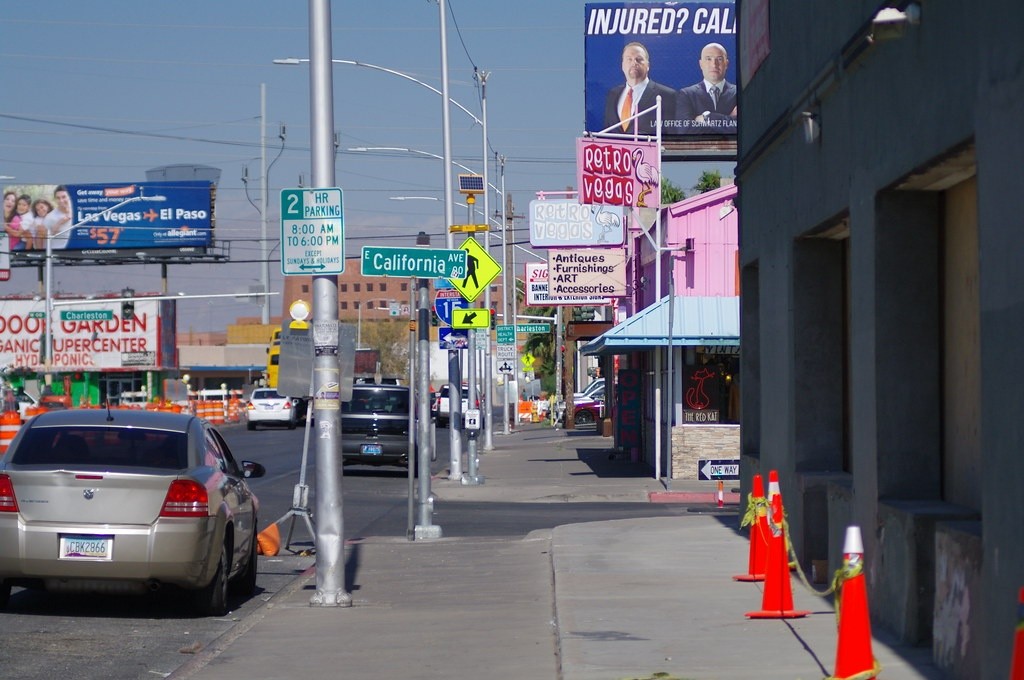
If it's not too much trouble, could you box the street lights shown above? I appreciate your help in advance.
[44,195,168,363]
[272,57,511,451]
[357,297,396,349]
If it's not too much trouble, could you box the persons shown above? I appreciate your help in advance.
[606,42,677,135]
[542,407,558,425]
[3,384,63,412]
[4,185,73,249]
[677,42,738,134]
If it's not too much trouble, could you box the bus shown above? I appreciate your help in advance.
[265,327,282,389]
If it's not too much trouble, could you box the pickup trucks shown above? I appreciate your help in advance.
[436,384,480,429]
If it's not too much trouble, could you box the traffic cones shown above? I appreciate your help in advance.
[743,494,811,618]
[732,471,797,581]
[833,527,876,680]
[1010,587,1024,680]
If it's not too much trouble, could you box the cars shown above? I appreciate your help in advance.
[561,401,605,424]
[0,410,268,615]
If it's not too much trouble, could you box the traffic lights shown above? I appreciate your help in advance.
[587,306,596,320]
[574,308,582,321]
[491,307,497,330]
[432,306,438,326]
[562,345,565,368]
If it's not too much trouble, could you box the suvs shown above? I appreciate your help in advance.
[544,378,606,422]
[245,387,299,430]
[342,383,419,478]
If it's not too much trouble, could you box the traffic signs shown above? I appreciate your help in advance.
[697,458,741,482]
[281,187,346,276]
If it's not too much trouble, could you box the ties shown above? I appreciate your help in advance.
[710,86,719,111]
[620,89,633,132]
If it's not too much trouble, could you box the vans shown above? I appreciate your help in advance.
[356,378,401,412]
